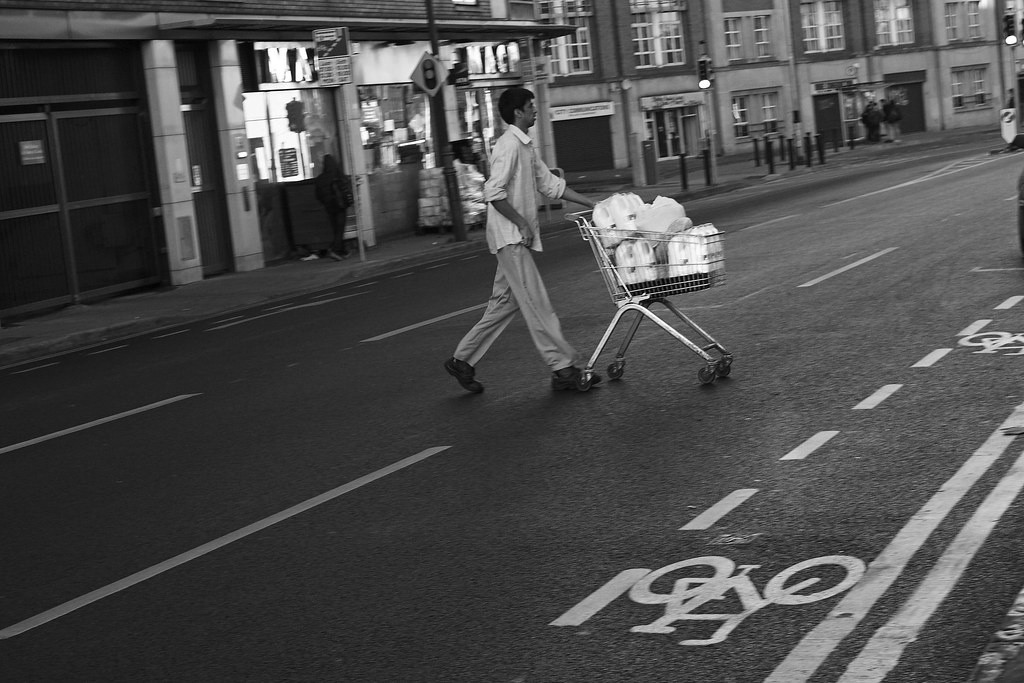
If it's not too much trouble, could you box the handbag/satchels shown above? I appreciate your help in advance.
[346,192,354,207]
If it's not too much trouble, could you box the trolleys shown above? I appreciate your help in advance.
[563,204,736,396]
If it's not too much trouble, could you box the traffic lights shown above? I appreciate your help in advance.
[411,51,450,98]
[706,59,717,84]
[1020,18,1024,37]
[1007,15,1016,37]
[697,59,708,89]
[1003,15,1010,38]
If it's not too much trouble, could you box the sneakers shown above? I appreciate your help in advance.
[444,357,484,391]
[552,369,603,391]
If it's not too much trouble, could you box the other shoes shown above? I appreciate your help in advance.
[331,252,344,260]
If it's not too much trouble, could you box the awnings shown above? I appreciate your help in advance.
[158,14,578,40]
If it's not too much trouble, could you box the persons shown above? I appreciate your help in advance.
[1008,89,1015,108]
[861,99,900,142]
[445,89,602,391]
[315,154,350,259]
